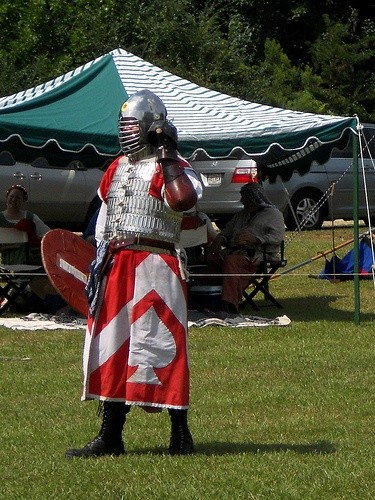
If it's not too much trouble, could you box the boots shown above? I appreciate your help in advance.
[66,402,127,457]
[169,408,194,454]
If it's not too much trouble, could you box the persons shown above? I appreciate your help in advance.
[213,181,284,314]
[65,88,202,458]
[180,209,222,266]
[84,208,97,249]
[0,184,60,301]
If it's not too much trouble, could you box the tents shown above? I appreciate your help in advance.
[0,47,361,326]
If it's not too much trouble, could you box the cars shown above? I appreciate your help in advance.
[260,122,375,232]
[0,151,261,236]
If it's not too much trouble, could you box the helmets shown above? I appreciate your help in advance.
[117,89,168,157]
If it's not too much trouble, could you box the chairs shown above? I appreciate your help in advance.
[0,219,288,316]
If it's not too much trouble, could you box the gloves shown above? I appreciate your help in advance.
[148,119,177,160]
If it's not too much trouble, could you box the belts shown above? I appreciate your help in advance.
[135,237,175,252]
